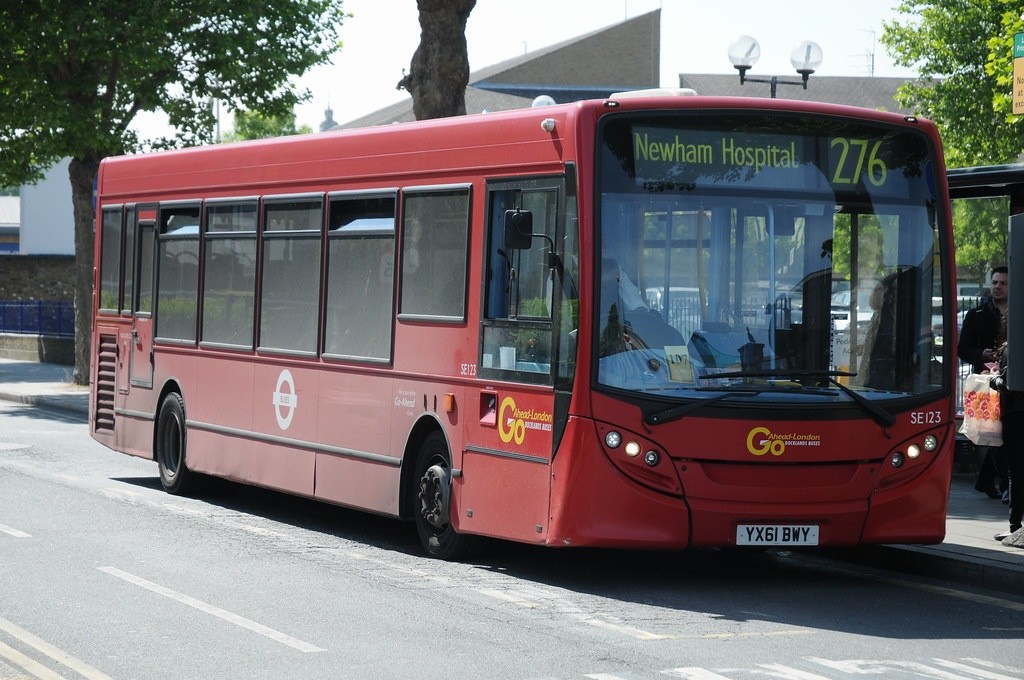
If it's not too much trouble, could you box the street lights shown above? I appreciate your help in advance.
[726,33,824,98]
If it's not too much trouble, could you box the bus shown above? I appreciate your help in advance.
[88,91,959,560]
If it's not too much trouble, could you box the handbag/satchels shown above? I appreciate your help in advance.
[958,374,1002,446]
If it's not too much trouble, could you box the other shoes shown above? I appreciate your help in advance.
[1002,490,1009,504]
[995,529,1012,540]
[974,479,1003,498]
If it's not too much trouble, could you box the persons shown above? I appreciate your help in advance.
[957,267,1024,541]
[865,272,896,391]
[545,224,650,318]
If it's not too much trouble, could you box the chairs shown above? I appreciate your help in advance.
[167,250,239,267]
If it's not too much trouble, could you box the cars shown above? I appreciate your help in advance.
[644,283,981,383]
